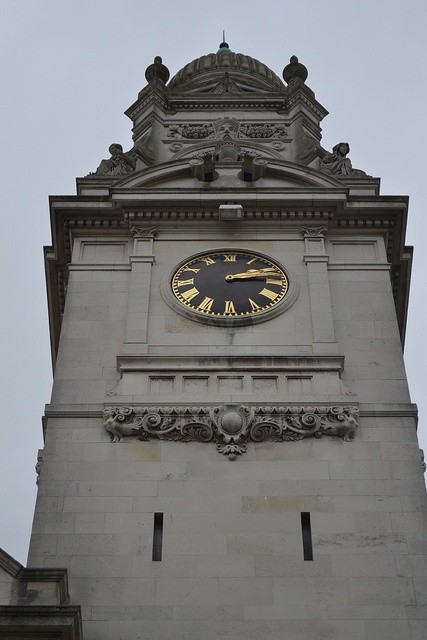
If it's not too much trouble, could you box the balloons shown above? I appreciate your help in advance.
[159,247,300,327]
